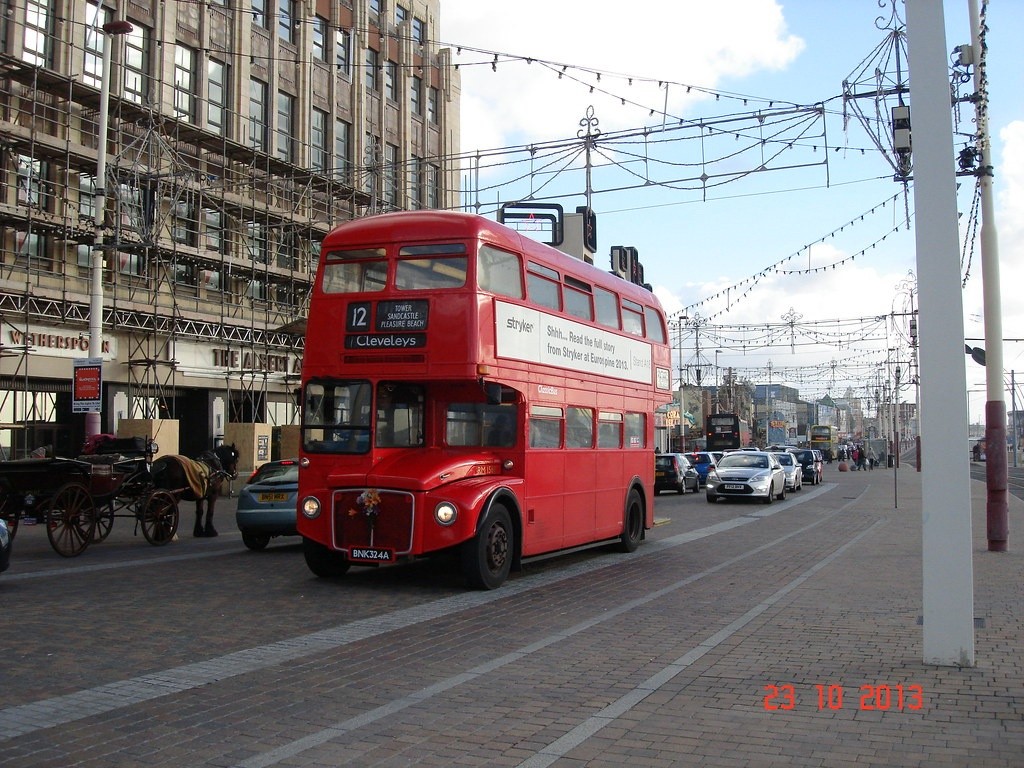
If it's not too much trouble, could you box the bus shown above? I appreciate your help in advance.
[297,200,670,592]
[809,425,839,462]
[706,413,750,450]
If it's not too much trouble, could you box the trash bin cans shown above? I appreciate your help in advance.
[888,453,895,468]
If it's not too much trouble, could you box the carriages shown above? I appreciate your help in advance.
[1,435,240,560]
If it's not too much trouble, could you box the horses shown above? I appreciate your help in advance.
[151,441,239,539]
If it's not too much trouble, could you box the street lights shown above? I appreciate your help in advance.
[678,315,689,452]
[888,346,900,466]
[765,386,778,448]
[715,349,724,414]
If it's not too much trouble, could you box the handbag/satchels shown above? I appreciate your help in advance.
[865,459,869,466]
[874,460,879,466]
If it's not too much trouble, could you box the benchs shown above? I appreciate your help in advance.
[0,457,93,482]
[78,453,124,478]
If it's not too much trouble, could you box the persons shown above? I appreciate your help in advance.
[825,445,877,472]
[655,447,661,454]
[487,408,617,448]
[694,445,700,452]
[972,444,979,462]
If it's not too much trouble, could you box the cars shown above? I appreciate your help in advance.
[713,443,824,486]
[681,453,718,487]
[237,457,299,550]
[705,451,787,505]
[770,452,804,493]
[653,451,701,495]
